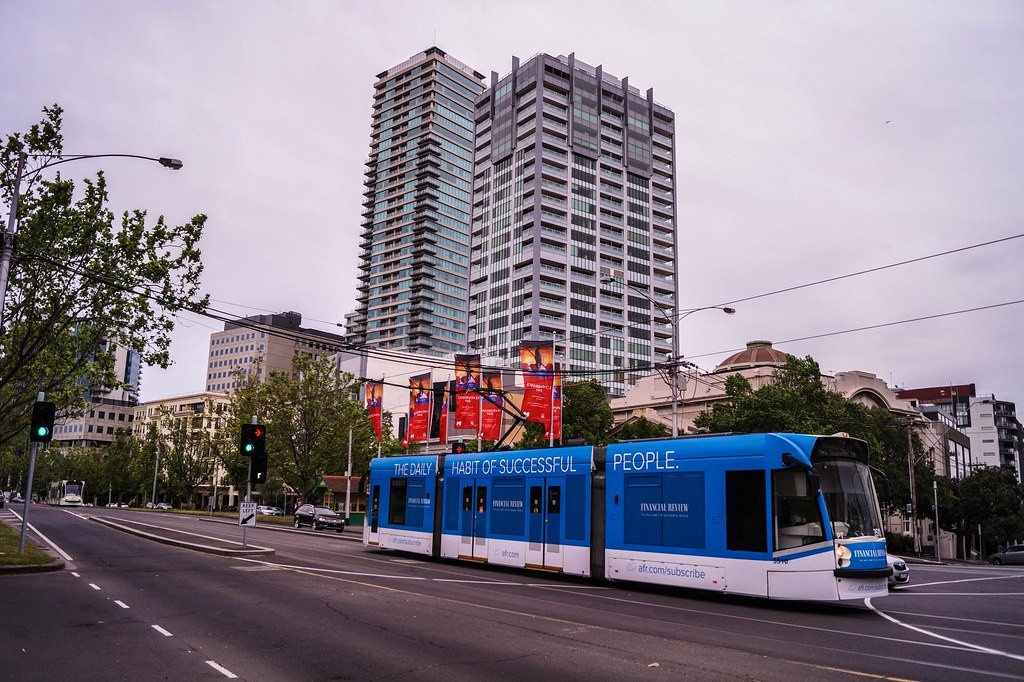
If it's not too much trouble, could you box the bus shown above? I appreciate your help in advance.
[362,431,889,601]
[47,479,84,506]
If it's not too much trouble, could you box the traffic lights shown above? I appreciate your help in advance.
[30,402,56,442]
[241,425,266,457]
[452,442,467,453]
[251,455,266,483]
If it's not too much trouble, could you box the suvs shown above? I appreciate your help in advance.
[294,504,344,533]
[0,490,5,508]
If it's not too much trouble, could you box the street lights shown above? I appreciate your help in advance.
[0,154,183,324]
[599,277,736,436]
[147,438,159,502]
[909,399,996,558]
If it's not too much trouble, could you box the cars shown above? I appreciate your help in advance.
[256,505,284,517]
[106,503,115,507]
[115,501,129,508]
[156,503,172,510]
[988,544,1024,566]
[146,502,156,508]
[887,554,910,587]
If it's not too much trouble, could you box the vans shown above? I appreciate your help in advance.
[11,497,25,504]
